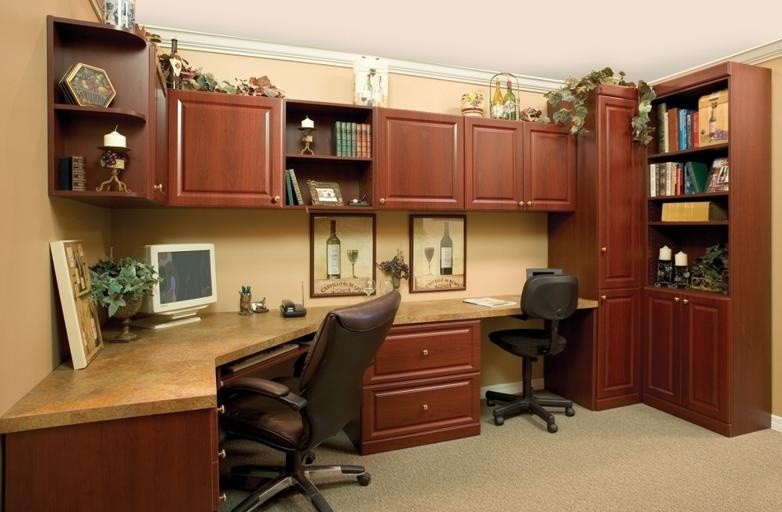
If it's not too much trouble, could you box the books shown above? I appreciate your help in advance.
[367,124,371,158]
[686,162,708,194]
[286,170,295,206]
[677,169,684,195]
[362,124,367,158]
[659,103,666,153]
[357,124,362,157]
[659,163,666,196]
[290,169,305,206]
[650,164,657,198]
[687,114,692,149]
[694,111,699,148]
[684,166,695,195]
[656,163,659,196]
[334,121,342,156]
[347,123,351,157]
[341,122,347,157]
[668,108,679,152]
[664,112,669,153]
[463,297,517,309]
[352,123,357,157]
[354,58,389,107]
[672,163,684,196]
[679,109,697,151]
[666,162,672,196]
[691,116,694,148]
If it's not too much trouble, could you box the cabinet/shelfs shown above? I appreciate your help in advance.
[463,116,578,213]
[47,14,168,209]
[547,84,648,411]
[642,61,774,438]
[167,87,465,212]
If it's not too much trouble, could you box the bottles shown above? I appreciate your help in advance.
[326,220,341,279]
[708,110,717,138]
[439,221,453,275]
[504,80,518,121]
[164,38,182,90]
[490,81,506,121]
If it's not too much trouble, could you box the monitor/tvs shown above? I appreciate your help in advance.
[133,240,218,330]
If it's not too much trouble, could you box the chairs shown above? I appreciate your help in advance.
[215,288,402,512]
[484,274,579,433]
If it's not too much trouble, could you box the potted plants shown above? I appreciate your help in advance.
[89,255,164,345]
[378,250,409,289]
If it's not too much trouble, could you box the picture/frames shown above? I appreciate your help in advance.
[307,179,344,206]
[408,214,467,294]
[309,212,376,299]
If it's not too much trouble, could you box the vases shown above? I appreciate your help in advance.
[461,108,485,118]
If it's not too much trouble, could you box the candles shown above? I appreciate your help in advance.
[674,250,688,266]
[658,245,672,260]
[300,115,314,129]
[103,123,126,147]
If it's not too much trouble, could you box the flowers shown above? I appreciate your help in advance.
[460,92,485,108]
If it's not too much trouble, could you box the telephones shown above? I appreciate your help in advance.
[279,280,307,318]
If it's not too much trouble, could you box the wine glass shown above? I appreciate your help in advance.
[424,247,435,277]
[362,279,378,302]
[380,279,392,295]
[347,249,359,278]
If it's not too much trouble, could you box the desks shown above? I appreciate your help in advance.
[0,293,599,512]
